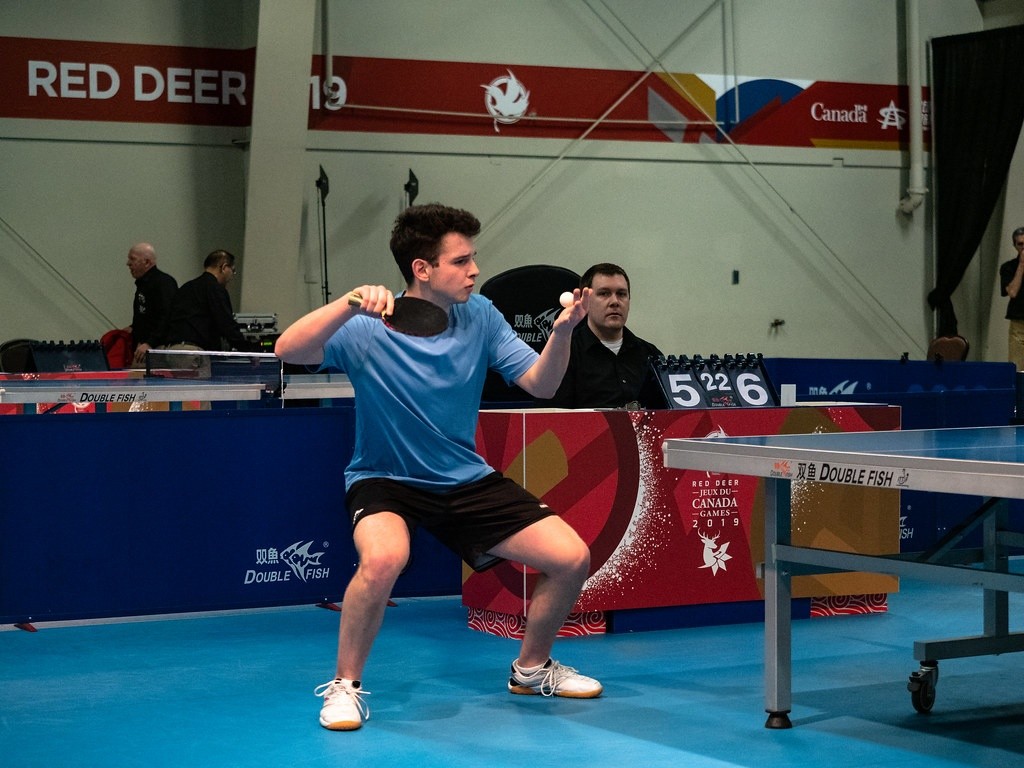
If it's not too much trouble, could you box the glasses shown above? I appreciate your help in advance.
[220,263,236,275]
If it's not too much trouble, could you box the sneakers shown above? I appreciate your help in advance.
[313,677,372,731]
[507,656,603,700]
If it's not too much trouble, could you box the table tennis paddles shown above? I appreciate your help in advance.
[346,293,452,337]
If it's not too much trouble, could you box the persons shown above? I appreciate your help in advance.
[998,226,1024,375]
[177,249,267,378]
[118,242,180,370]
[551,262,672,408]
[272,200,604,733]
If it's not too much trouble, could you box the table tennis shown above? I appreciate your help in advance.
[561,291,575,308]
